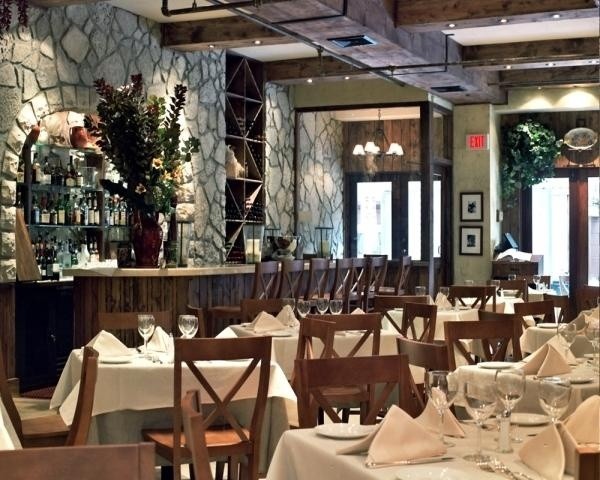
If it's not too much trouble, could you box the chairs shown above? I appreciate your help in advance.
[291,354,407,428]
[185,306,203,335]
[98,310,172,341]
[273,254,600,379]
[0,402,157,480]
[239,298,280,319]
[3,375,71,448]
[208,260,279,329]
[141,334,273,478]
[396,335,470,422]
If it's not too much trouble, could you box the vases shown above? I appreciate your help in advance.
[133,217,162,269]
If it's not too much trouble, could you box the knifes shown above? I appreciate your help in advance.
[364,455,454,471]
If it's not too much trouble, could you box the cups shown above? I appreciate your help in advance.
[508,274,517,281]
[539,377,574,419]
[177,312,199,338]
[414,285,426,296]
[295,297,346,318]
[463,279,501,291]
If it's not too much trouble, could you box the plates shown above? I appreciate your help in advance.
[478,360,512,369]
[504,410,548,429]
[314,423,371,440]
[566,374,593,384]
[101,355,131,365]
[564,127,597,151]
[538,322,557,328]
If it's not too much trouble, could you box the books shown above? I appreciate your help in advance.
[496,248,532,263]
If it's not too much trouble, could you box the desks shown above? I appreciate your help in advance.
[48,343,300,478]
[222,317,411,405]
[450,359,599,421]
[265,417,598,480]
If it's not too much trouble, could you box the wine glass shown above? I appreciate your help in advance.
[137,314,155,351]
[282,297,297,331]
[464,374,497,466]
[439,286,450,311]
[495,367,531,444]
[532,274,542,291]
[422,366,456,450]
[557,318,600,372]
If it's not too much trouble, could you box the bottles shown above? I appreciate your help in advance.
[31,152,84,187]
[225,115,264,222]
[15,190,102,226]
[103,196,129,227]
[29,235,100,280]
[226,246,244,263]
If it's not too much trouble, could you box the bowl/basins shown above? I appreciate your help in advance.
[266,235,301,257]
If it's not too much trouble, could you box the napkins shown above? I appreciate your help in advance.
[83,330,131,360]
[518,424,581,478]
[245,311,281,330]
[335,405,445,468]
[146,327,173,351]
[561,393,598,446]
[213,328,237,340]
[415,396,465,440]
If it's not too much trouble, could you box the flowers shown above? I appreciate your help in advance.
[84,72,203,216]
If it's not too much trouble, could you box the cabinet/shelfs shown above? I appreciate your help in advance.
[224,47,267,264]
[24,143,133,277]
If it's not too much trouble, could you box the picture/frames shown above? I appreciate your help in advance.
[458,226,484,257]
[460,192,485,221]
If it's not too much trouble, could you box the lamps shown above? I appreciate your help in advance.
[350,107,405,156]
